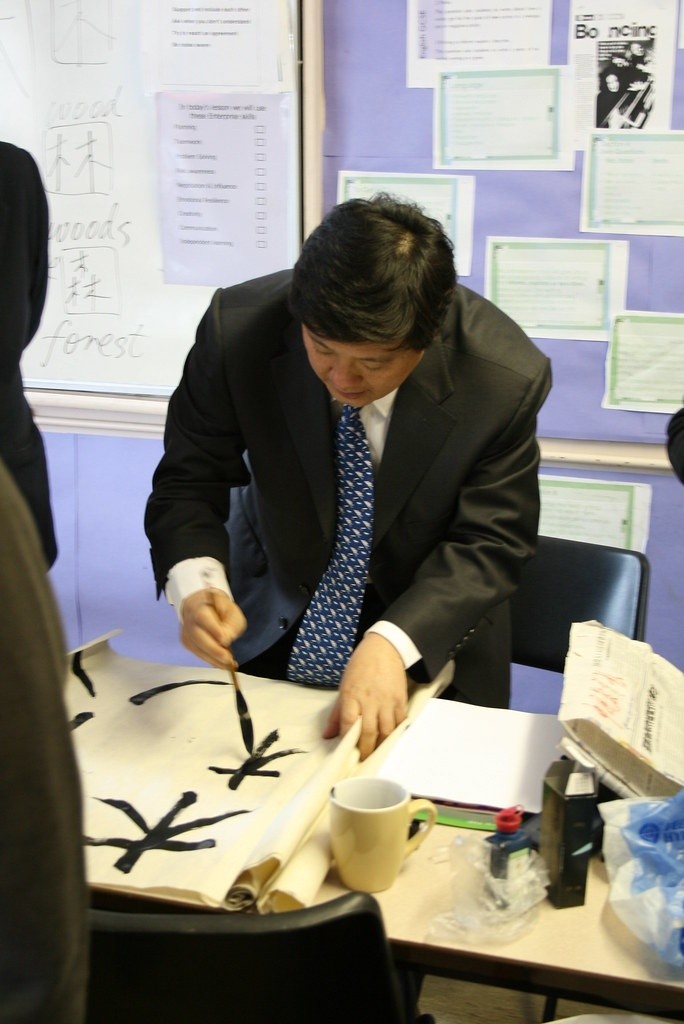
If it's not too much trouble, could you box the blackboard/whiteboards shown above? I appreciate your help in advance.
[0,0,327,422]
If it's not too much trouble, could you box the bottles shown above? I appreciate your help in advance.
[478,805,532,910]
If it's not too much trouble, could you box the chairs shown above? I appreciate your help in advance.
[83,889,436,1024]
[510,535,651,674]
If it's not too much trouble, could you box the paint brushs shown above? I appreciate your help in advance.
[201,570,254,756]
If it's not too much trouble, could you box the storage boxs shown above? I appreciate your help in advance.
[537,737,599,910]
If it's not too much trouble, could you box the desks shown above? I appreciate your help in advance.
[83,802,684,1024]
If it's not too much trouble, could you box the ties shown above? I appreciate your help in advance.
[287,404,373,688]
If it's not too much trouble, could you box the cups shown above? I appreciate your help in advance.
[330,778,438,894]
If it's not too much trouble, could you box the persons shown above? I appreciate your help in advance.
[0,140,58,574]
[595,41,656,131]
[0,463,87,1024]
[143,198,553,764]
